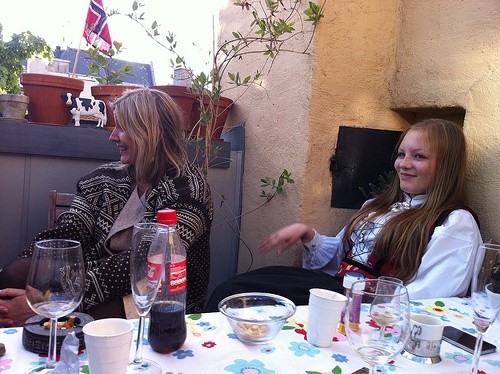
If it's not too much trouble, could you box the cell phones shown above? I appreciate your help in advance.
[442,326,497,356]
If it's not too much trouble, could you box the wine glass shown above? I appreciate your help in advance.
[130,223,169,374]
[25,239,85,374]
[345,276,411,374]
[460,242,500,374]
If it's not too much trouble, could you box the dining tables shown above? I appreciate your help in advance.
[0,296,500,374]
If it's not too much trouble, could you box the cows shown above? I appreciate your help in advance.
[60,91,108,129]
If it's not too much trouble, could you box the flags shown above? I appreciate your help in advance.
[84,0,112,54]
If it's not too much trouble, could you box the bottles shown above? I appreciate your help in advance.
[146,209,187,354]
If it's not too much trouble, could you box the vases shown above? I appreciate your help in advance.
[18,72,83,126]
[148,85,232,141]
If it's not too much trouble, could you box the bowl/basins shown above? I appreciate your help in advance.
[218,292,296,344]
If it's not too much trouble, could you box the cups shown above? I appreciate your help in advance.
[307,288,348,347]
[82,318,133,374]
[404,314,444,357]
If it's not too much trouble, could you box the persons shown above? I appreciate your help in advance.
[1,87,214,328]
[206,119,486,312]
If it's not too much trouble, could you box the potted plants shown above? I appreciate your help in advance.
[0,18,54,125]
[87,44,145,130]
